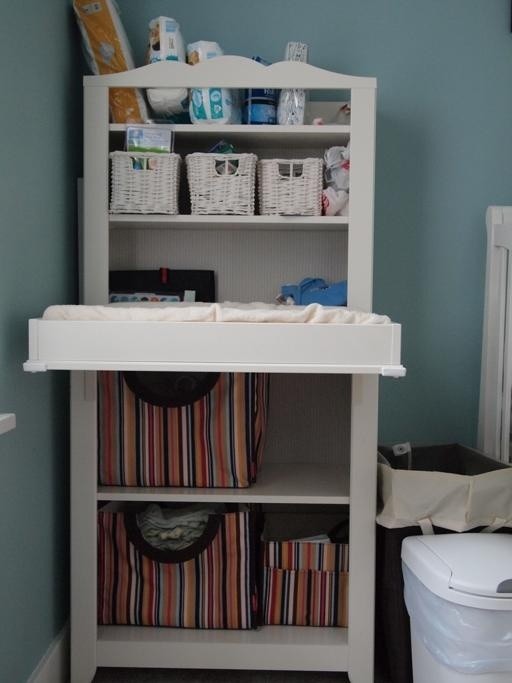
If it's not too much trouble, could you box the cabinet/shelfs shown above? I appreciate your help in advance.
[26,58,402,683]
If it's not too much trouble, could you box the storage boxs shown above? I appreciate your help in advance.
[260,537,348,627]
[99,501,264,634]
[99,371,268,489]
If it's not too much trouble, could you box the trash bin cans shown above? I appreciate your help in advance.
[401,533,512,683]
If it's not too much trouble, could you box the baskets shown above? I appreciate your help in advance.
[260,157,324,217]
[112,150,179,215]
[187,152,255,214]
